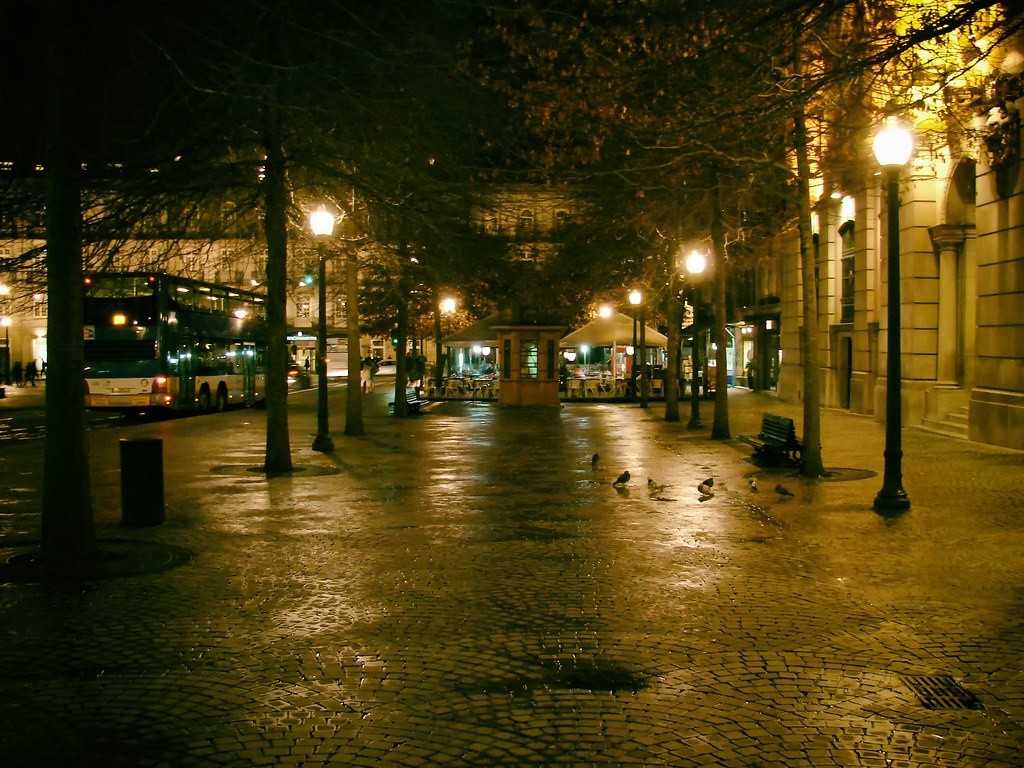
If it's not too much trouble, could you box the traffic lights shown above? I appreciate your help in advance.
[305,276,313,284]
[392,337,399,345]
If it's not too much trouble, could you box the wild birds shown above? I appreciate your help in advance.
[704,478,714,487]
[591,454,599,465]
[698,483,714,495]
[648,477,657,489]
[613,471,630,485]
[649,485,665,499]
[748,475,757,491]
[776,484,795,498]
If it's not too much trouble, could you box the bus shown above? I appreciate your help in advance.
[82,269,265,416]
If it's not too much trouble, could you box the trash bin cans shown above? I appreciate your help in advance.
[120,438,165,524]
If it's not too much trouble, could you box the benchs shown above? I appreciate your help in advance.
[737,412,822,467]
[388,390,430,413]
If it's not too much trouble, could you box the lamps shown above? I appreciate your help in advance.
[625,342,634,356]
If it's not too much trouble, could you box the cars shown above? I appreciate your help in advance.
[286,362,311,389]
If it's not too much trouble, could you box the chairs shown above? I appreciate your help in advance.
[420,377,501,400]
[565,379,664,399]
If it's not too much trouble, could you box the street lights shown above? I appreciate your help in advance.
[2,318,12,385]
[308,206,335,451]
[685,249,707,431]
[869,114,914,516]
[444,298,455,377]
[629,291,641,402]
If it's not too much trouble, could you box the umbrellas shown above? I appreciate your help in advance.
[559,311,666,346]
[435,305,513,348]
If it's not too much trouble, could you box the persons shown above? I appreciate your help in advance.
[562,358,653,397]
[303,356,310,372]
[406,352,427,387]
[12,358,40,388]
[359,349,393,395]
